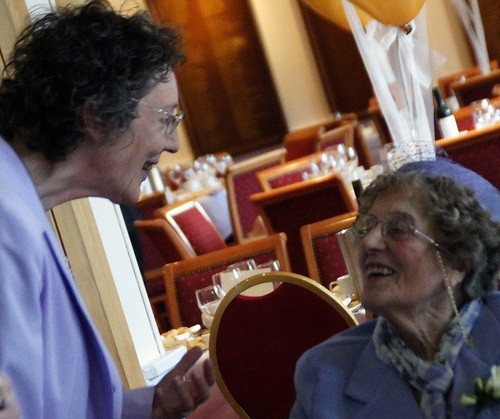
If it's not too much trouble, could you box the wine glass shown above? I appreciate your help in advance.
[168,155,232,200]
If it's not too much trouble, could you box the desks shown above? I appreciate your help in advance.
[174,175,232,241]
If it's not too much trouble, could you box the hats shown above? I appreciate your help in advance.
[396,158,500,224]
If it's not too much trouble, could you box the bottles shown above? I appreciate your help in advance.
[352,179,364,197]
[433,86,459,139]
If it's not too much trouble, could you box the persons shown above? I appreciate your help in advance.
[288,168,500,419]
[0,0,216,419]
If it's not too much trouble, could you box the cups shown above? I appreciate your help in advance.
[472,98,500,128]
[194,259,281,315]
[329,273,356,295]
[303,143,362,192]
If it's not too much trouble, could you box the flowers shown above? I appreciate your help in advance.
[462,365,500,419]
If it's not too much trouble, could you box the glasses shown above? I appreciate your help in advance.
[129,96,185,136]
[353,211,459,263]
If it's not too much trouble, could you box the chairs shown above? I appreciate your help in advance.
[125,61,500,419]
